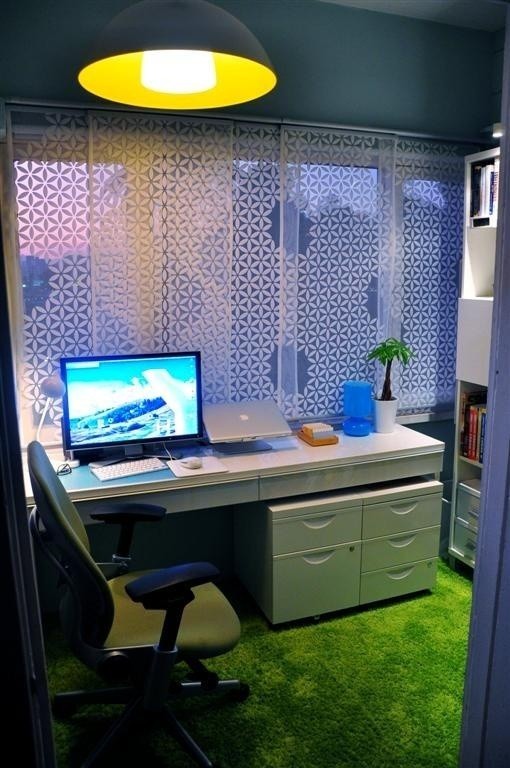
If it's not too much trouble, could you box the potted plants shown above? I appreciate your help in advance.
[367,337,414,434]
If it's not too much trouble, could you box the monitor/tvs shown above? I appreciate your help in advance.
[60,351,203,468]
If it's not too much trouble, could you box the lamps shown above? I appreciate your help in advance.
[75,1,278,110]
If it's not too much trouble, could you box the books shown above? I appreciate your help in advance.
[459,392,487,463]
[471,158,500,217]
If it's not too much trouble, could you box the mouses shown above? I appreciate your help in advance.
[180,457,202,469]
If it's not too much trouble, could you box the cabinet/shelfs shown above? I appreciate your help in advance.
[447,147,501,570]
[360,483,444,604]
[272,500,364,624]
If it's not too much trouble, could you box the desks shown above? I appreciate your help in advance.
[21,422,444,530]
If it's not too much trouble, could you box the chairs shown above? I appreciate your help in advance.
[26,440,249,768]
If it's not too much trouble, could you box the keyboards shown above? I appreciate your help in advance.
[89,456,170,483]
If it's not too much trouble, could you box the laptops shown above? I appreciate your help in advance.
[202,399,293,444]
